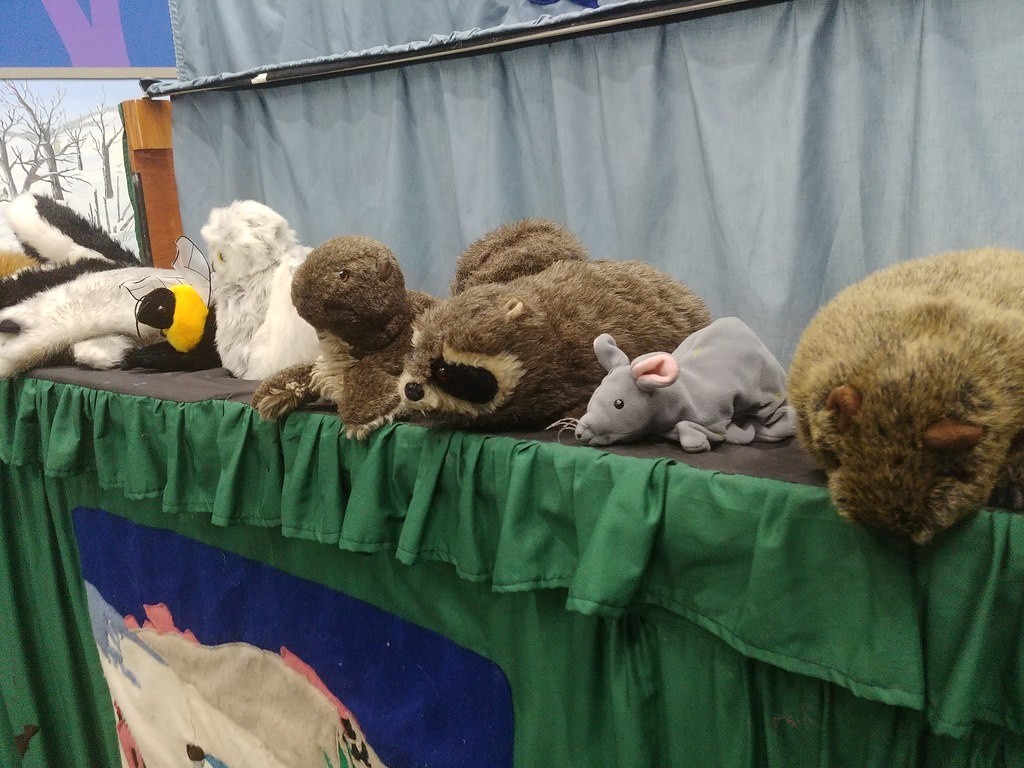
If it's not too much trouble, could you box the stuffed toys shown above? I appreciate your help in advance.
[121,283,223,373]
[547,316,795,453]
[397,258,714,434]
[201,200,321,382]
[788,246,1023,546]
[0,193,219,381]
[252,219,583,441]
